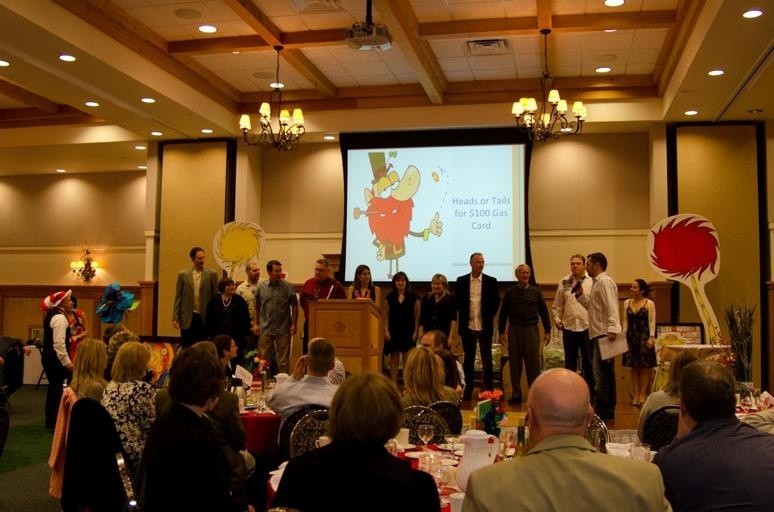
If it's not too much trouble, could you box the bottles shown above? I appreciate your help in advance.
[512,426,525,457]
[256,396,266,413]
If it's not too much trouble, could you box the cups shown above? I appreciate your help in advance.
[397,428,410,446]
[627,441,651,461]
[314,436,330,447]
[448,492,465,512]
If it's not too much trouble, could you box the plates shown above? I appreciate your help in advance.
[401,443,515,467]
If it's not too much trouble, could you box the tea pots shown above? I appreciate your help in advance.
[229,377,248,406]
[455,428,498,491]
[273,373,290,390]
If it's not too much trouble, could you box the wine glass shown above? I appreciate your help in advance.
[444,433,459,460]
[419,425,433,449]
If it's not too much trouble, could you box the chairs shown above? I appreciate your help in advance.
[57,388,140,511]
[643,401,683,453]
[586,411,611,450]
[276,396,464,463]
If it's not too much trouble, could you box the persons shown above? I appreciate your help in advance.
[552,254,597,401]
[461,368,672,512]
[498,264,550,406]
[623,279,657,406]
[43,288,467,510]
[651,359,774,512]
[572,252,622,420]
[637,352,700,444]
[172,247,454,388]
[454,253,501,399]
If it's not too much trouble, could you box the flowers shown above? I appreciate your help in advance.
[92,281,141,334]
[477,387,507,436]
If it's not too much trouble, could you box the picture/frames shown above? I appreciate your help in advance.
[653,322,704,366]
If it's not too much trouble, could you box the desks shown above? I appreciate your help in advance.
[21,344,49,386]
[229,381,282,458]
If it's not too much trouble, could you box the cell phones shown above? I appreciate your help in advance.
[244,406,256,410]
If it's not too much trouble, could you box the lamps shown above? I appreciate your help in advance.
[235,45,307,153]
[68,245,98,283]
[507,26,587,142]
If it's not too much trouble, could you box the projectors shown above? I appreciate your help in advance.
[344,25,393,53]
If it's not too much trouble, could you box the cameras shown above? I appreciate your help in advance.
[224,376,242,388]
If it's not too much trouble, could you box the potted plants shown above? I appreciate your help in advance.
[717,294,759,394]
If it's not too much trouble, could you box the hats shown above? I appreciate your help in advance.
[40,289,72,313]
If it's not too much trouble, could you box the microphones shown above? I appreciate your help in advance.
[571,275,586,293]
[328,279,336,286]
